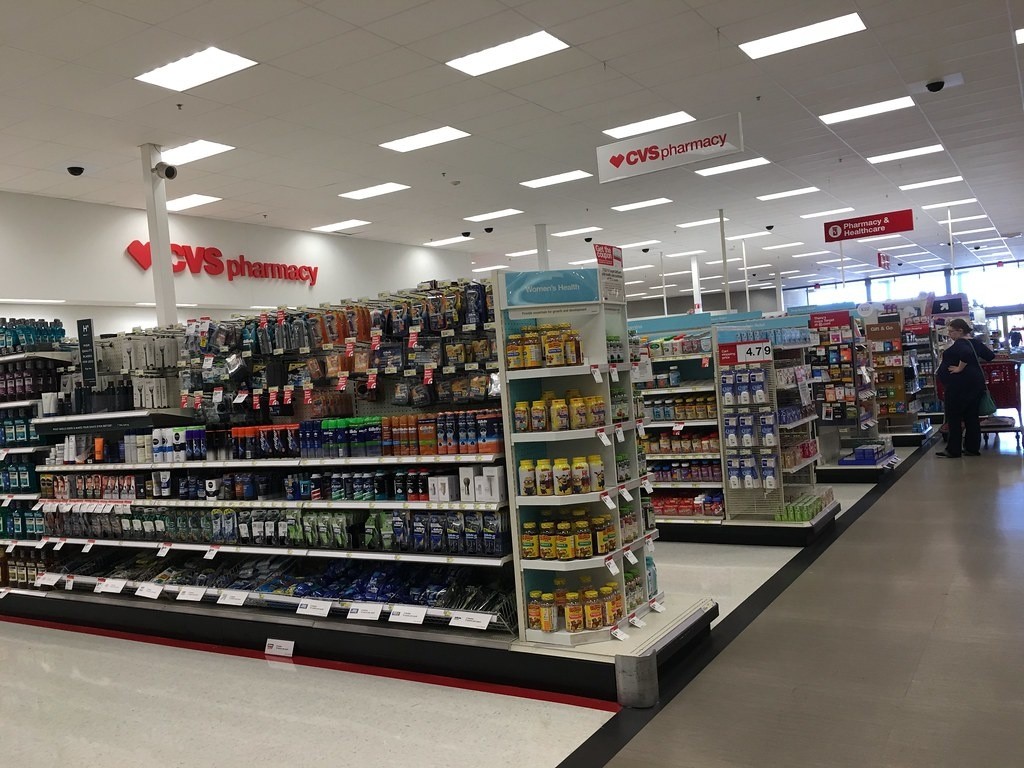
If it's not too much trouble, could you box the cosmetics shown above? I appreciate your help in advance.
[178,473,268,500]
[42,392,58,417]
[57,377,135,417]
[151,471,171,499]
[45,426,206,465]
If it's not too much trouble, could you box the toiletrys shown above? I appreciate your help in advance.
[0,317,516,636]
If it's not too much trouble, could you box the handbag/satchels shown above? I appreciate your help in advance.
[978,390,996,416]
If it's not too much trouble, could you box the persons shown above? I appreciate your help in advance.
[1008,326,1023,347]
[53,476,132,494]
[935,319,996,458]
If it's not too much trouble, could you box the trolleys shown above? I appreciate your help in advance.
[934,354,1024,448]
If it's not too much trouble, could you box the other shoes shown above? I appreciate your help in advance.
[935,452,951,458]
[961,449,981,456]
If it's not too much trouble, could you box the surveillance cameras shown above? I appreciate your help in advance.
[642,249,649,253]
[462,232,470,237]
[67,167,84,176]
[766,225,773,230]
[485,228,493,233]
[898,263,902,266]
[753,274,756,276]
[974,246,980,250]
[926,81,945,93]
[585,238,592,242]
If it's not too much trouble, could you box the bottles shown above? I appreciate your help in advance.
[522,506,615,560]
[553,577,570,616]
[643,396,722,483]
[514,387,606,434]
[518,454,606,497]
[283,465,448,500]
[118,408,502,461]
[578,575,592,598]
[620,496,657,547]
[565,592,584,632]
[528,590,542,630]
[615,444,647,484]
[606,330,642,363]
[601,586,617,626]
[624,556,658,615]
[606,582,624,619]
[541,593,558,633]
[507,320,585,374]
[611,387,644,422]
[585,590,603,631]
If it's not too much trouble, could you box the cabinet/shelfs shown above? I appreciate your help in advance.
[626,302,945,528]
[0,264,716,664]
